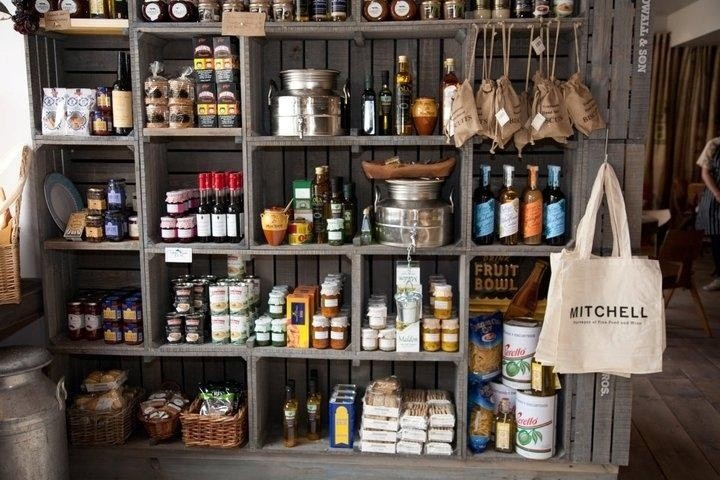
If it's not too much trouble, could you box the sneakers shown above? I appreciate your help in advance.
[702,279,720,291]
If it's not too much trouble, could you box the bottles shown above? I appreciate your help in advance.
[282,377,300,447]
[304,368,325,444]
[112,51,134,135]
[196,169,246,243]
[497,165,520,248]
[493,396,516,454]
[360,208,374,246]
[505,259,550,319]
[472,163,497,248]
[543,163,567,247]
[394,55,414,138]
[378,69,394,137]
[440,56,458,133]
[520,165,542,248]
[360,75,378,136]
[310,162,357,248]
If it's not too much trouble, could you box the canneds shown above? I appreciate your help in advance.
[362,274,458,351]
[66,293,137,348]
[420,0,575,18]
[87,186,138,242]
[289,221,312,235]
[515,388,558,460]
[326,218,344,245]
[289,235,311,245]
[89,88,111,135]
[197,0,294,23]
[502,316,538,390]
[253,273,348,350]
[163,255,261,343]
[160,188,200,239]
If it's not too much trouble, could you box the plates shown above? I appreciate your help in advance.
[42,171,86,235]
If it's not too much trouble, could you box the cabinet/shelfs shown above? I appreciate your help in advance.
[11,0,586,465]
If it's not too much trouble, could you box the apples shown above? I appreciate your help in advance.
[518,429,531,445]
[506,361,519,376]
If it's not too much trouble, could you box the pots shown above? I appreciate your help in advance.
[266,65,352,138]
[374,177,457,249]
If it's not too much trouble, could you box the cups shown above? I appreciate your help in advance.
[409,95,440,136]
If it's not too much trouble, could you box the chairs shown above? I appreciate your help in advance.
[657,228,714,339]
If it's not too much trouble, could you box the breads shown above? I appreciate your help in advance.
[138,389,190,419]
[70,370,133,413]
[146,75,194,129]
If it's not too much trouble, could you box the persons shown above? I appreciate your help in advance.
[695,138,720,292]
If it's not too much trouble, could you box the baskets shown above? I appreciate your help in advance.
[137,390,190,441]
[67,388,145,447]
[0,229,23,306]
[179,395,247,449]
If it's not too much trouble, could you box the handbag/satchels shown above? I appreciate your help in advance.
[532,162,667,380]
